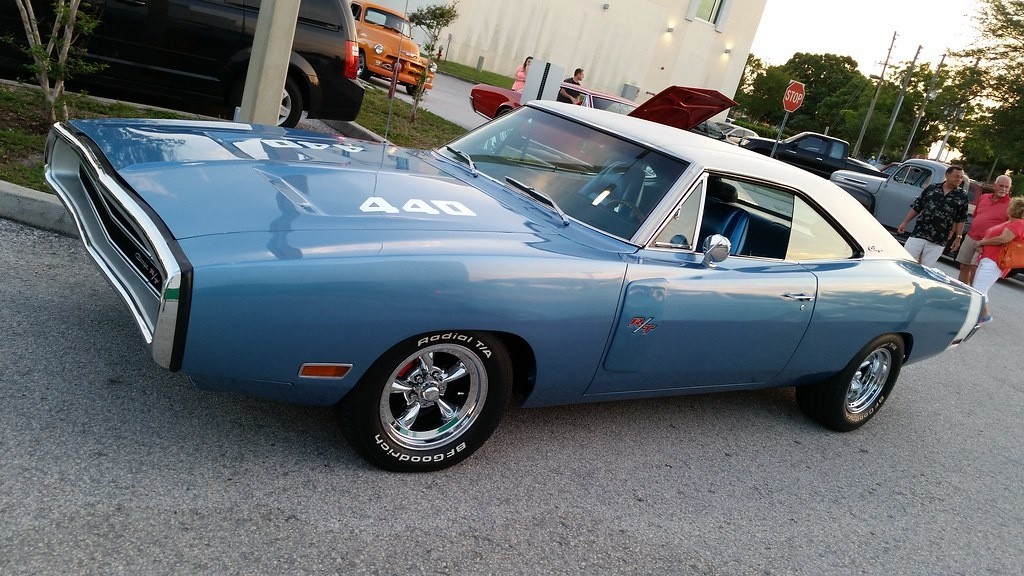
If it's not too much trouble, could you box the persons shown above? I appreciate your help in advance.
[972,196,1024,323]
[897,166,968,268]
[511,56,534,92]
[955,175,1012,287]
[557,68,585,105]
[867,156,877,166]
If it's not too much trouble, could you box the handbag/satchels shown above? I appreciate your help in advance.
[997,241,1024,269]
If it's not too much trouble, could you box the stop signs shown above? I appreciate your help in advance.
[783,81,806,112]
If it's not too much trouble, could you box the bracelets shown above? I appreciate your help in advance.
[977,243,981,246]
[957,234,962,238]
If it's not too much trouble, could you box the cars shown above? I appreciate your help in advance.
[346,1,438,101]
[689,119,739,146]
[468,85,741,170]
[714,121,760,146]
[41,98,996,473]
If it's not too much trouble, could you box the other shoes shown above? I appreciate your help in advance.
[976,313,993,326]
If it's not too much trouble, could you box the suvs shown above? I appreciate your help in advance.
[0,1,366,128]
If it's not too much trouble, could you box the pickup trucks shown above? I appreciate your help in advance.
[738,131,881,182]
[828,158,1024,278]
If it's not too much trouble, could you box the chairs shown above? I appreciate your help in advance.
[579,157,650,211]
[700,185,753,253]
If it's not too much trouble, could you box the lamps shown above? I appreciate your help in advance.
[668,27,674,33]
[724,47,732,55]
[603,3,610,10]
